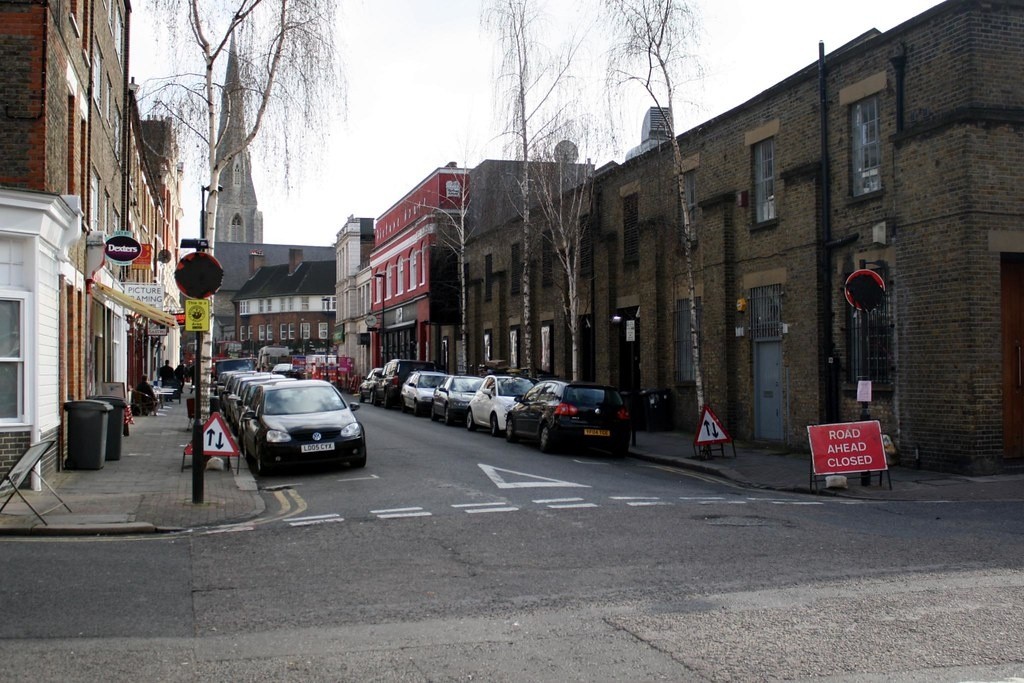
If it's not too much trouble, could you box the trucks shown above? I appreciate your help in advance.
[257,346,290,371]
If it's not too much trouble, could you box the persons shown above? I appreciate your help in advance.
[159,360,174,402]
[188,360,196,394]
[174,360,187,395]
[136,374,158,416]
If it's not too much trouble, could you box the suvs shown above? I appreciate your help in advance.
[214,358,259,398]
[373,359,440,408]
[400,370,449,415]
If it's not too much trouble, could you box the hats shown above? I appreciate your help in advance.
[188,360,192,362]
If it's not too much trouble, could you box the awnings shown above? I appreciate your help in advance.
[95,282,179,330]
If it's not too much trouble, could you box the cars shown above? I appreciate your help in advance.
[431,375,485,425]
[506,380,631,458]
[239,379,366,475]
[271,364,305,378]
[359,367,385,401]
[217,369,297,429]
[465,376,539,436]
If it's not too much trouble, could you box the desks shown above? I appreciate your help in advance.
[152,388,177,408]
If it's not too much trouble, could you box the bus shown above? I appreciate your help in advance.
[184,342,243,376]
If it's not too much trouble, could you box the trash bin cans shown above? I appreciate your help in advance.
[85,395,126,462]
[61,399,114,471]
[640,388,673,431]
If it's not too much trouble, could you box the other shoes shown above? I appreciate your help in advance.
[169,400,174,402]
[165,399,168,402]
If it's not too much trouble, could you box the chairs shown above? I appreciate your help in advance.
[132,390,157,416]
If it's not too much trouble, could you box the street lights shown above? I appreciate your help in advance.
[322,298,329,381]
[376,273,386,368]
[202,184,225,248]
